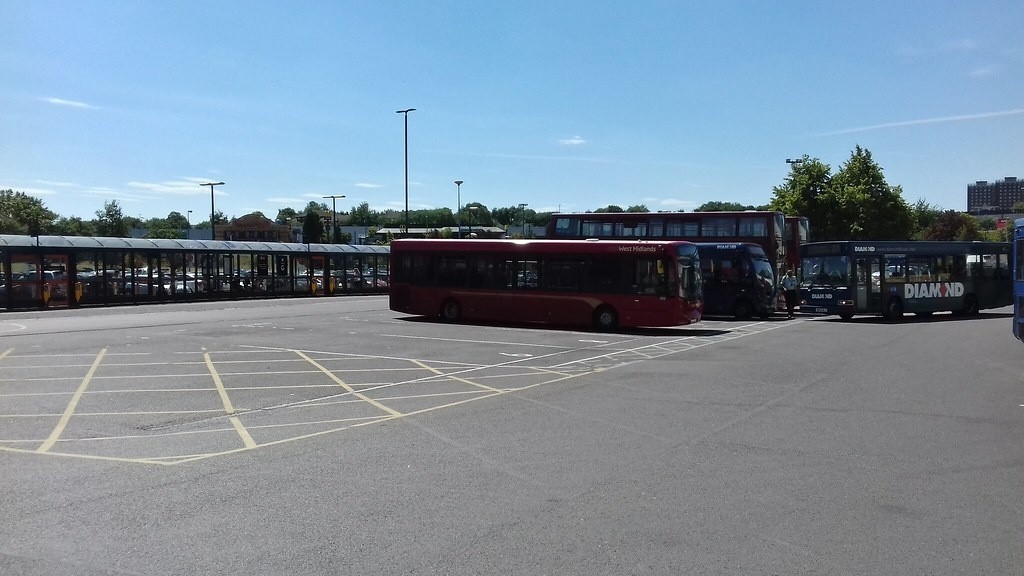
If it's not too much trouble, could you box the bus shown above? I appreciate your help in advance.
[1010,218,1024,343]
[389,237,706,332]
[797,239,1015,323]
[780,216,809,291]
[536,240,779,320]
[548,209,785,289]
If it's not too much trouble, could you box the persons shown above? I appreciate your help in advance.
[829,263,841,279]
[780,270,797,318]
[113,270,121,278]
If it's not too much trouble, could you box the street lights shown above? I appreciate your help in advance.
[518,202,529,284]
[469,206,479,237]
[198,182,226,241]
[322,194,346,243]
[187,210,194,238]
[395,107,417,237]
[454,181,464,239]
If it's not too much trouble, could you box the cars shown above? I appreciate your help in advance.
[0,266,389,301]
[872,265,910,283]
